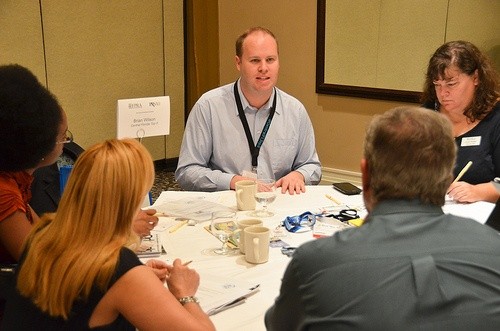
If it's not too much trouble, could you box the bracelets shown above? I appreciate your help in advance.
[178,296,199,304]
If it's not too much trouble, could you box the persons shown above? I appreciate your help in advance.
[265,106,500,331]
[175,27,322,195]
[420,41,500,205]
[0,64,159,263]
[0,137,216,331]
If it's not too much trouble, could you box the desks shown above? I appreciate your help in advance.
[124,185,495,331]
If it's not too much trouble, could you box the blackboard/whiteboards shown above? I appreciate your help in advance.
[316,0,500,104]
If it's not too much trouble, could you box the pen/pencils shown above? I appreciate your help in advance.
[170,220,188,232]
[152,213,164,217]
[326,194,340,205]
[183,261,192,266]
[453,161,473,182]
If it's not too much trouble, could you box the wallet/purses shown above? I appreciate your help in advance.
[332,182,361,195]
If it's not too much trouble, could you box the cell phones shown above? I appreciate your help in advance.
[332,182,362,195]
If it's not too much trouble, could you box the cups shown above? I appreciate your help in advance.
[243,226,270,264]
[237,218,262,255]
[235,180,258,212]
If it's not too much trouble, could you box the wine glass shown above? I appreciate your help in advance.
[256,179,278,217]
[211,210,238,255]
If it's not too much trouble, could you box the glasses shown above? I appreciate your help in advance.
[55,130,73,144]
